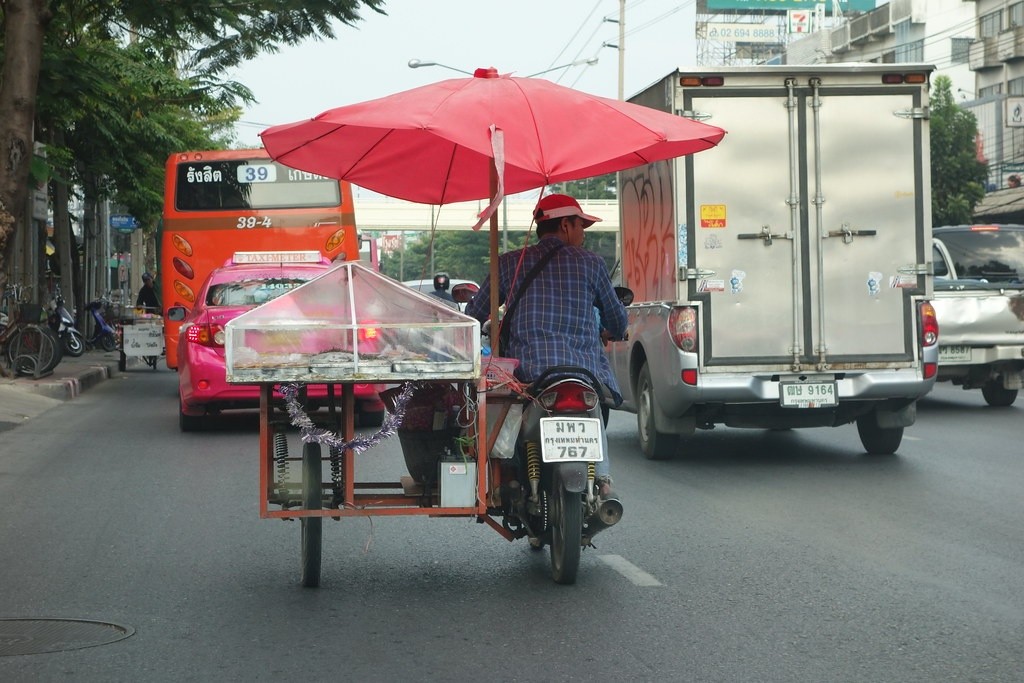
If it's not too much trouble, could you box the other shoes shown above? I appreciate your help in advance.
[476,486,503,507]
[600,483,619,500]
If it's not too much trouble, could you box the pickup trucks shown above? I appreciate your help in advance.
[929,238,1024,407]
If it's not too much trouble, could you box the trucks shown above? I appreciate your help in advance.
[590,59,939,463]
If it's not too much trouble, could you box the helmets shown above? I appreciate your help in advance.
[434,275,449,289]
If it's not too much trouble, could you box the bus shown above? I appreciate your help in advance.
[162,146,361,371]
[358,234,380,272]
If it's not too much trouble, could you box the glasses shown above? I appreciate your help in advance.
[144,278,150,281]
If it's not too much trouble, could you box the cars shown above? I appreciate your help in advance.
[930,223,1024,289]
[176,258,388,431]
[397,276,483,314]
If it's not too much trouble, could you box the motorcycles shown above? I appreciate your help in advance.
[451,260,636,588]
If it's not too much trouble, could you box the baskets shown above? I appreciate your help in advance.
[481,359,520,396]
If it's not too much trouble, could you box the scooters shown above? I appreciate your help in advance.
[80,300,117,352]
[42,283,85,357]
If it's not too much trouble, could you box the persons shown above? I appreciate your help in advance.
[133,273,160,309]
[465,194,627,508]
[428,274,456,305]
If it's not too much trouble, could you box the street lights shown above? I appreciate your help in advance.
[403,56,599,247]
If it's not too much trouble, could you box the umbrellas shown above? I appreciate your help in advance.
[257,68,725,358]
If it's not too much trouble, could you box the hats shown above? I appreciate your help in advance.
[142,273,154,283]
[533,194,602,229]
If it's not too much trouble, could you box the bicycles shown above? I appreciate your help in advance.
[117,304,166,372]
[102,302,122,350]
[0,281,64,379]
